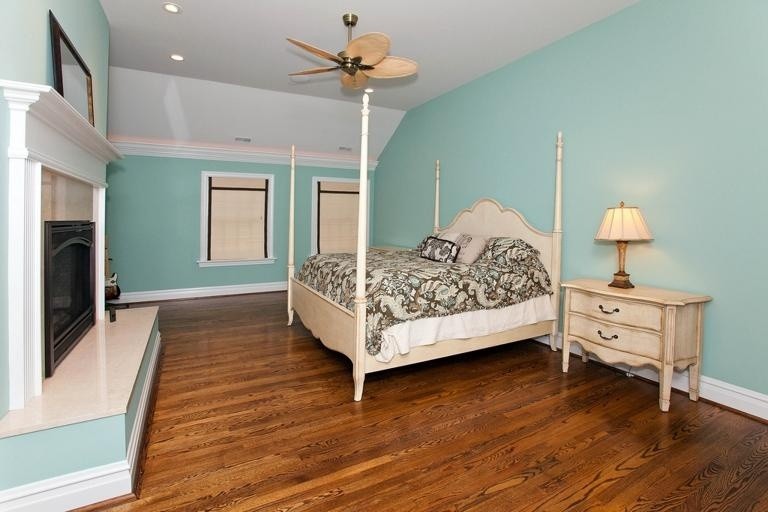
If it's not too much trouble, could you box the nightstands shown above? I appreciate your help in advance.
[562,278,713,413]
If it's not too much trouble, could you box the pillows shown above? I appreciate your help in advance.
[437,228,489,265]
[420,235,461,263]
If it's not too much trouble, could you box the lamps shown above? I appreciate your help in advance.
[594,201,654,290]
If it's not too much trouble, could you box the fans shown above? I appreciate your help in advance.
[286,13,418,89]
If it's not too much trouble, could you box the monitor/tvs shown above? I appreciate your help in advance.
[44,220,96,377]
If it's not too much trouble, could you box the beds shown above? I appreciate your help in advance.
[286,92,564,402]
